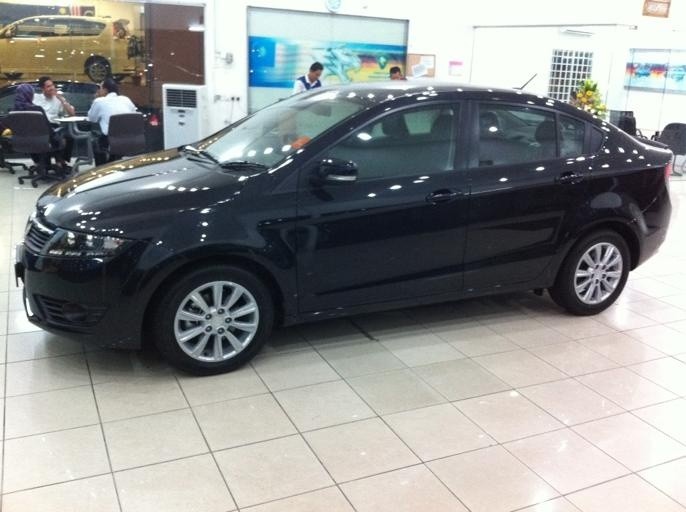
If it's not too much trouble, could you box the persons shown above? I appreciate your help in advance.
[88,79,138,168]
[31,76,77,175]
[388,66,408,81]
[293,62,324,96]
[16,83,81,176]
[92,80,104,99]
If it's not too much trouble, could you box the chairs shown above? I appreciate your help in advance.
[607,110,686,176]
[479,112,565,162]
[374,113,456,175]
[0,112,152,187]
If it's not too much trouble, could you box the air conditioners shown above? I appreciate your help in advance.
[161,83,210,150]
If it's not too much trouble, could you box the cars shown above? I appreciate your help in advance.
[0,10,141,86]
[8,73,680,384]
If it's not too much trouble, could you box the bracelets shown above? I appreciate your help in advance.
[61,100,67,105]
